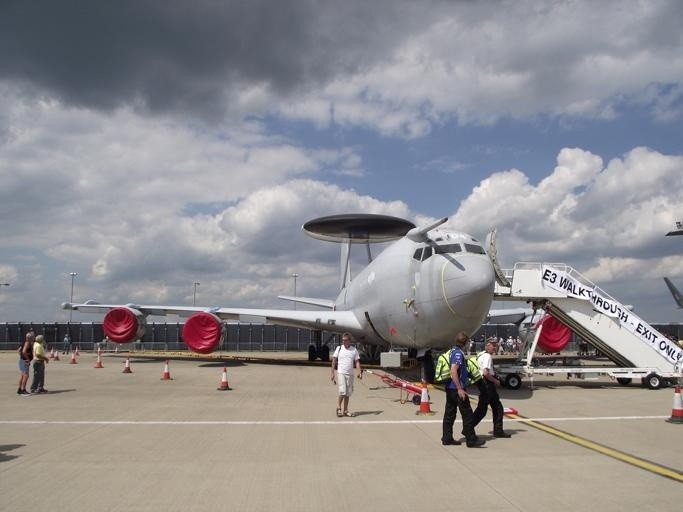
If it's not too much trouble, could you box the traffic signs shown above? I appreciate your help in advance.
[192,281,201,305]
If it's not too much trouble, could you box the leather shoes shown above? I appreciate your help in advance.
[443,440,461,445]
[494,432,510,437]
[466,439,485,447]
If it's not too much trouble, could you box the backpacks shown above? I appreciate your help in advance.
[466,352,489,384]
[435,349,455,381]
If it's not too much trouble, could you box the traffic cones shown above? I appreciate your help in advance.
[94,351,104,369]
[217,368,233,391]
[664,383,682,424]
[49,344,80,364]
[121,358,133,374]
[416,378,435,416]
[160,360,174,381]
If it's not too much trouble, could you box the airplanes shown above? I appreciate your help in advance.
[61,207,634,383]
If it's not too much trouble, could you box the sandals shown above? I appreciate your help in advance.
[337,409,353,417]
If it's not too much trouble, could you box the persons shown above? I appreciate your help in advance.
[660,330,683,347]
[14,331,34,395]
[59,332,72,356]
[461,335,515,438]
[31,334,50,394]
[575,330,610,357]
[441,332,485,447]
[458,332,525,355]
[329,331,364,419]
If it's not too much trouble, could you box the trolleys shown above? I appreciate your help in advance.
[364,368,431,405]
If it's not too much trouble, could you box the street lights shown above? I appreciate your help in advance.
[291,273,300,309]
[0,283,9,287]
[66,271,78,321]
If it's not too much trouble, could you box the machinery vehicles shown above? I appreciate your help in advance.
[494,262,683,390]
